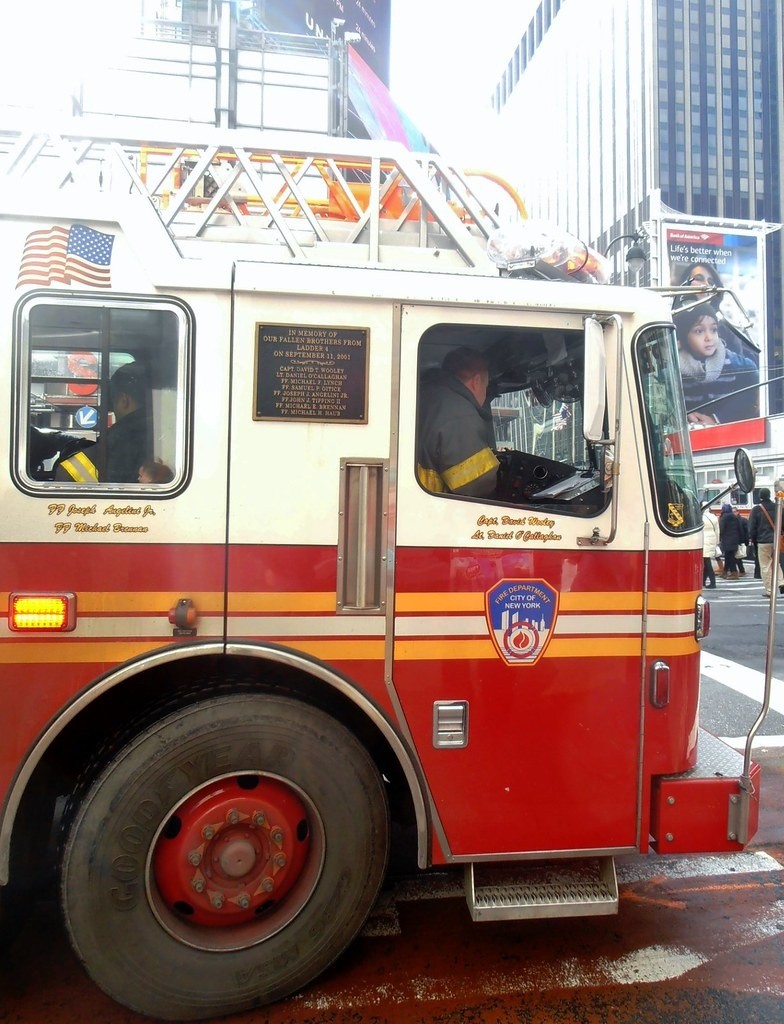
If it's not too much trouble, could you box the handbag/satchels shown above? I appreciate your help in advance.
[735,543,747,559]
[780,536,784,551]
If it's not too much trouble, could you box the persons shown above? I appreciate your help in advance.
[701,501,719,589]
[719,504,743,580]
[731,507,748,575]
[414,348,500,498]
[53,361,158,482]
[748,490,784,597]
[672,263,759,366]
[676,300,759,426]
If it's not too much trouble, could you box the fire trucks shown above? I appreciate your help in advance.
[1,109,783,1024]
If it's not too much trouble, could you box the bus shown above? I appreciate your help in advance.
[698,477,777,520]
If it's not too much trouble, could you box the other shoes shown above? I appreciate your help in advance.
[703,578,716,588]
[779,587,784,594]
[762,590,771,597]
[719,570,745,580]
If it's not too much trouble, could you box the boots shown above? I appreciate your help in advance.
[713,561,724,574]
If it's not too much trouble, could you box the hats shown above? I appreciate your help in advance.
[679,302,719,329]
[110,361,148,397]
[722,504,732,513]
[759,488,771,499]
